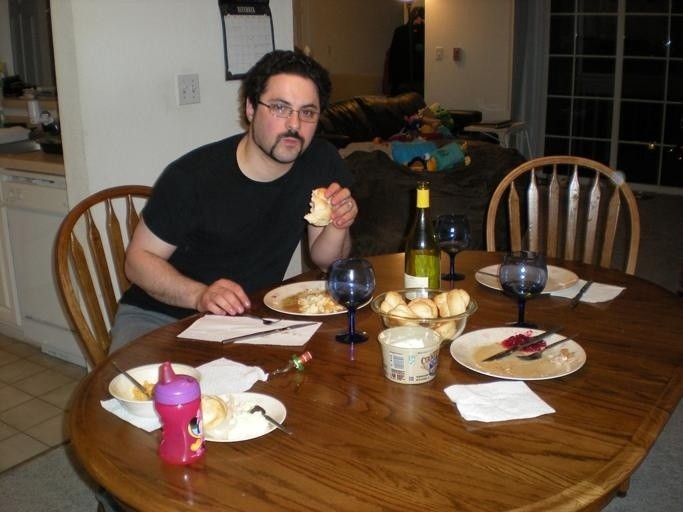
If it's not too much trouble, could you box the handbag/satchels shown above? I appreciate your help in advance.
[382,23,425,98]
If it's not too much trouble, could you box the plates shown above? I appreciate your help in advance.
[261,280,370,317]
[448,326,588,381]
[201,391,285,444]
[473,259,579,294]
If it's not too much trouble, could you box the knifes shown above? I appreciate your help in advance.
[569,276,594,309]
[219,322,319,347]
[483,326,565,361]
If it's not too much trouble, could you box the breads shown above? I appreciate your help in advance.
[304,189,335,227]
[380,288,471,340]
[201,394,227,430]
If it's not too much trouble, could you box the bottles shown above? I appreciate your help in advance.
[151,364,209,467]
[401,178,441,290]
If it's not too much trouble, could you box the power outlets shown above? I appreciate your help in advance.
[177,75,202,106]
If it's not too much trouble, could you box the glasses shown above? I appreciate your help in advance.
[257,100,321,124]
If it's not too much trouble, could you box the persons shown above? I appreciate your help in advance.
[106,47,363,353]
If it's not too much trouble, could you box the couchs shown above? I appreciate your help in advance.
[316,90,532,252]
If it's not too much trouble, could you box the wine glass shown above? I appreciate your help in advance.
[434,211,472,283]
[327,254,372,346]
[496,249,548,327]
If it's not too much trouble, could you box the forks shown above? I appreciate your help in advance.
[249,404,295,440]
[516,330,583,361]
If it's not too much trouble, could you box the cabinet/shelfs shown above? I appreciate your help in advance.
[0,168,88,368]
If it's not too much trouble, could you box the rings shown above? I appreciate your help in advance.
[346,199,354,210]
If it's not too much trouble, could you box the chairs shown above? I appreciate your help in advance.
[485,154,642,275]
[50,185,153,367]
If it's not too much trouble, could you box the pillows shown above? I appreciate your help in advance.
[392,141,437,166]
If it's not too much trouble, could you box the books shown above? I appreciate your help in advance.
[476,119,513,128]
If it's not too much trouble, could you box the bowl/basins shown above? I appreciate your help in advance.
[107,362,202,416]
[371,289,476,350]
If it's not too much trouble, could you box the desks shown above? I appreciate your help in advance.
[463,122,536,168]
